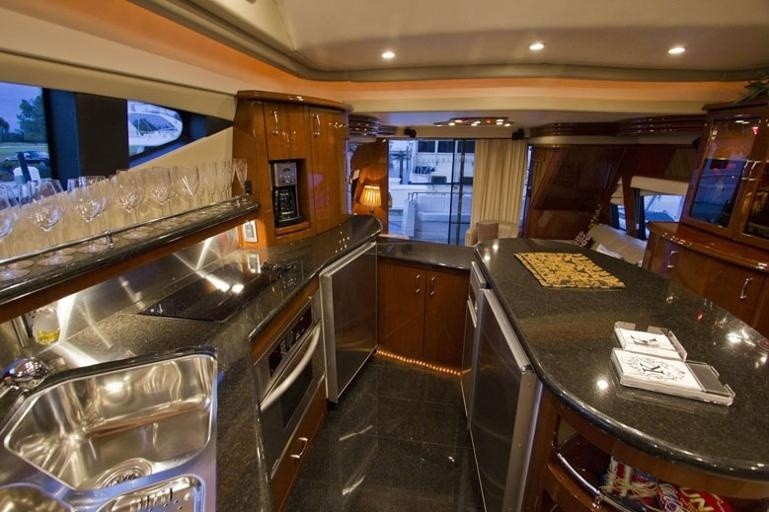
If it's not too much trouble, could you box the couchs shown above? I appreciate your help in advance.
[528,223,648,269]
[465,219,520,248]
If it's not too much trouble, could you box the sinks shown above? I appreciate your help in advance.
[0,346,218,492]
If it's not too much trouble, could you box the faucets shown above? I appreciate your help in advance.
[1,356,49,397]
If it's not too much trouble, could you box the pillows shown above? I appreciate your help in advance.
[476,223,498,242]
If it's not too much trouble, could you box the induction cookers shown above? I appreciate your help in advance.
[137,260,289,324]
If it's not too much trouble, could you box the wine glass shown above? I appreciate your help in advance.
[216,160,240,212]
[33,307,60,347]
[109,162,216,238]
[235,159,251,202]
[32,178,77,256]
[26,181,74,265]
[0,192,30,281]
[0,185,35,270]
[67,177,118,254]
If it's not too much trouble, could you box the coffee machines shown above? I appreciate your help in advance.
[272,161,305,228]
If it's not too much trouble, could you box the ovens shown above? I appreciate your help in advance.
[252,297,326,485]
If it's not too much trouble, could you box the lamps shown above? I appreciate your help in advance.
[358,185,382,216]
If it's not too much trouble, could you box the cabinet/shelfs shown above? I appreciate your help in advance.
[377,255,470,375]
[232,90,353,248]
[680,105,769,250]
[521,385,769,512]
[642,232,769,340]
